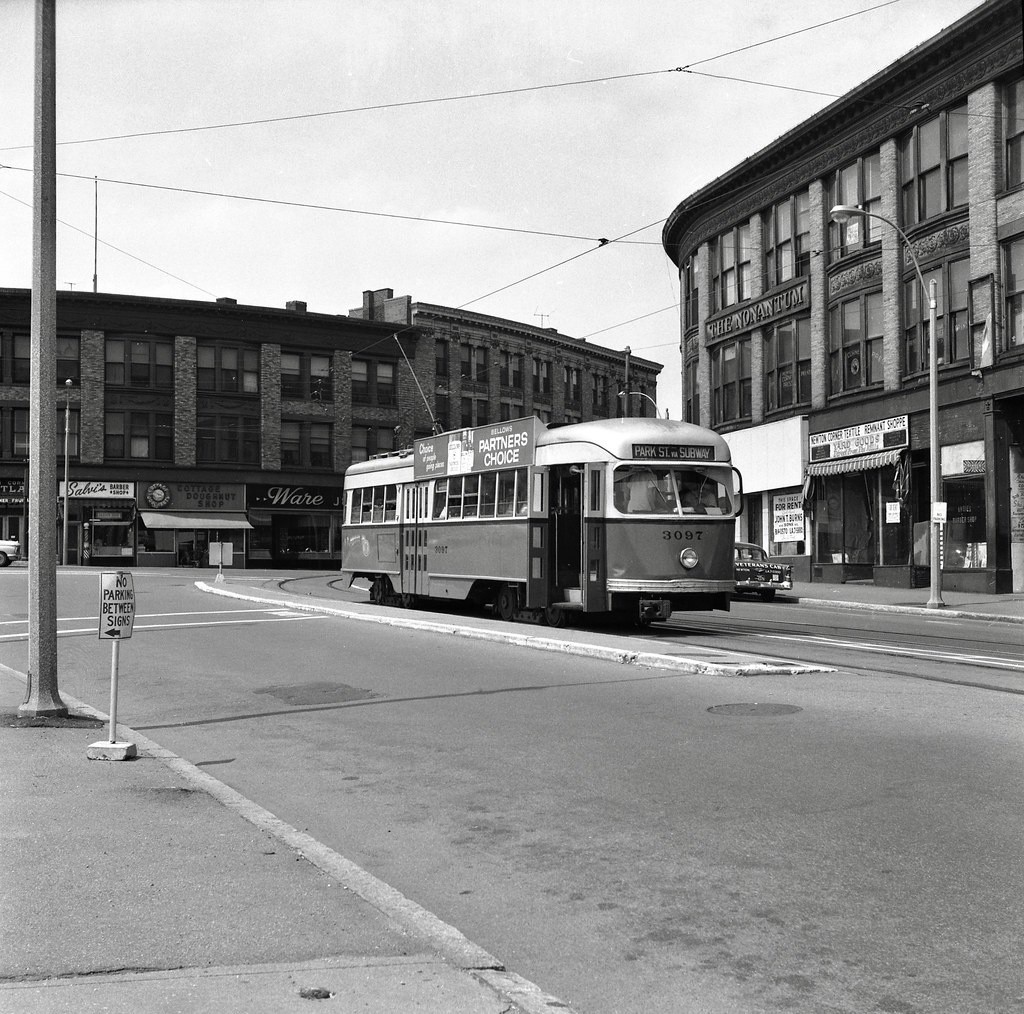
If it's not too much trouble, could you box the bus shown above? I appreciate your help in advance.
[340,332,743,632]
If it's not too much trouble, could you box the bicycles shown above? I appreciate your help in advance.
[180,549,209,568]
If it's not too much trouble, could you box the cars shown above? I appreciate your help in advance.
[0,539,22,568]
[733,542,794,603]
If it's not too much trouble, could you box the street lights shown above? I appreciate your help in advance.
[617,392,674,501]
[829,204,947,609]
[62,379,73,565]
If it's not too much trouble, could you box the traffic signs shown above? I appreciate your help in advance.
[98,572,136,639]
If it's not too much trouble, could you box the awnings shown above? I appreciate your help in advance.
[806,448,907,476]
[140,510,255,530]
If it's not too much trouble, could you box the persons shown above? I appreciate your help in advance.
[624,469,658,515]
[684,480,716,513]
